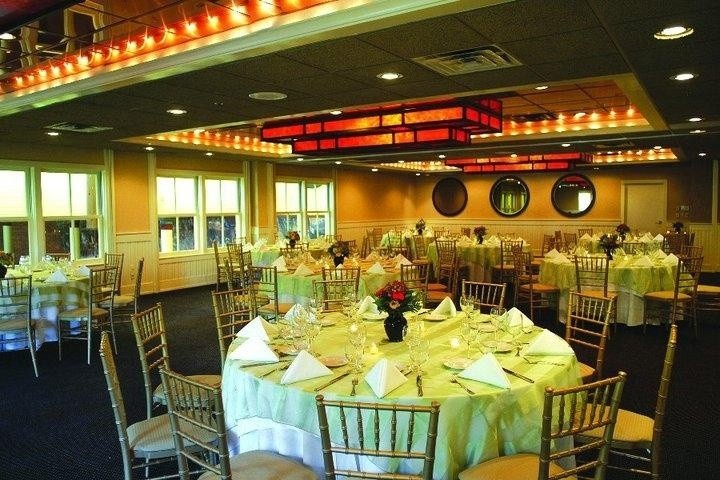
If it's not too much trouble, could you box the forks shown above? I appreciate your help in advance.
[449,378,475,395]
[350,378,360,397]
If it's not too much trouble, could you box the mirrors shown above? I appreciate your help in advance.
[432,177,468,216]
[551,174,597,218]
[490,175,530,217]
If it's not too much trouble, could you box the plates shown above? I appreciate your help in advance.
[441,355,472,368]
[423,313,448,322]
[316,354,350,367]
[364,309,382,321]
[278,340,304,355]
[489,339,513,355]
[317,317,335,327]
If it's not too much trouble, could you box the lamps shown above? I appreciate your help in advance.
[442,152,593,175]
[260,99,505,158]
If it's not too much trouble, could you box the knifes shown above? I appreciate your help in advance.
[502,368,534,384]
[417,374,423,396]
[315,373,348,391]
[238,360,289,369]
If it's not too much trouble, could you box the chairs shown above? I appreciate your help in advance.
[570,324,677,479]
[158,364,317,480]
[316,395,441,480]
[99,330,219,480]
[457,372,627,479]
[130,302,222,479]
[0,253,145,378]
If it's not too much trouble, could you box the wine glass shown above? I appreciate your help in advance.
[460,294,509,366]
[301,248,396,273]
[559,241,659,265]
[401,319,430,375]
[280,295,324,351]
[18,254,75,281]
[342,293,367,377]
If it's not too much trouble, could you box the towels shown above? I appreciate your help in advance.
[281,350,334,385]
[363,358,408,399]
[457,352,511,389]
